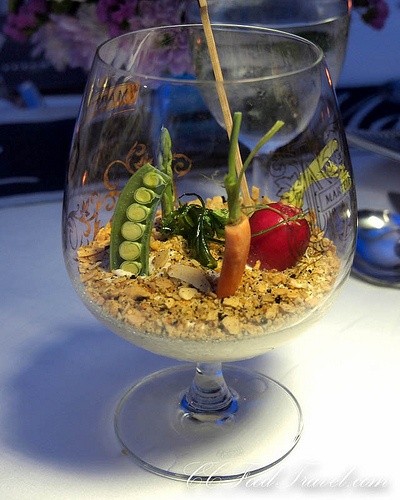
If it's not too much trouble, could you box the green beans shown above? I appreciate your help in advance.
[108,163,173,276]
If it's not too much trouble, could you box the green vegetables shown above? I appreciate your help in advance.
[155,192,228,269]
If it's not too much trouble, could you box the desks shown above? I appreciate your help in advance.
[2,128,399,500]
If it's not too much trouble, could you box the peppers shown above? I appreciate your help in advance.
[216,111,284,299]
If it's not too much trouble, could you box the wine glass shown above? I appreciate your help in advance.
[185,0,352,199]
[60,24,358,482]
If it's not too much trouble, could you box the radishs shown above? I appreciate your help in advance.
[248,138,351,272]
